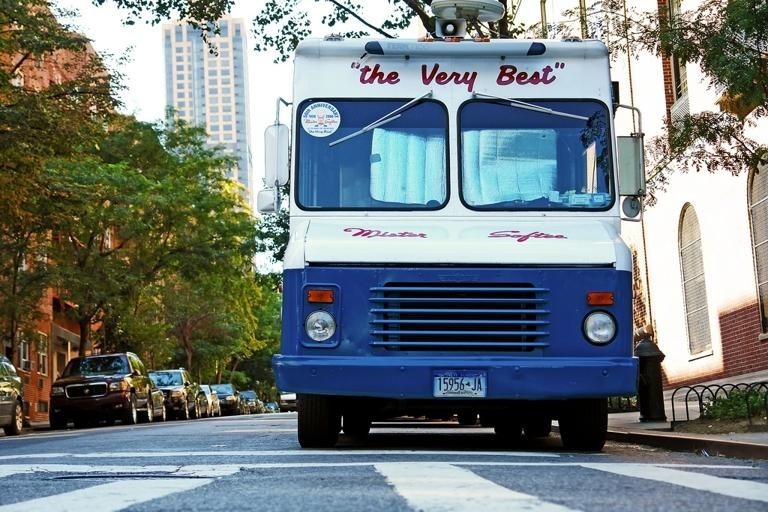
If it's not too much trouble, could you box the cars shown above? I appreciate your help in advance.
[0,352,24,436]
[151,377,166,422]
[239,390,279,414]
[194,381,208,417]
[199,385,222,416]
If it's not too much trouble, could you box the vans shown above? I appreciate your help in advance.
[280,390,298,412]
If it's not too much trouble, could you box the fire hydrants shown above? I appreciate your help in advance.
[633,330,666,422]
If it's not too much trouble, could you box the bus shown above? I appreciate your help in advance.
[255,1,645,453]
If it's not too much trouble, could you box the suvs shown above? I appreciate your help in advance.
[149,368,200,420]
[49,352,154,428]
[211,383,239,414]
